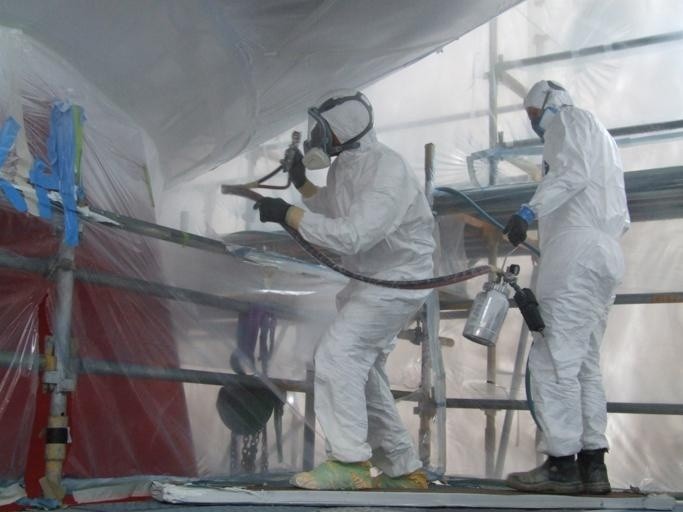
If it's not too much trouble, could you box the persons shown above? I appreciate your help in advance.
[498,78,631,496]
[249,86,442,493]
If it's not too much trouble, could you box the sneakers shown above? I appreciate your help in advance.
[372,470,430,490]
[290,458,372,491]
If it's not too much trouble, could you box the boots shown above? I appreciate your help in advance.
[506,454,584,494]
[577,448,611,494]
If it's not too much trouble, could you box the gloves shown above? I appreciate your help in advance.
[253,197,291,222]
[283,148,306,189]
[503,214,528,246]
